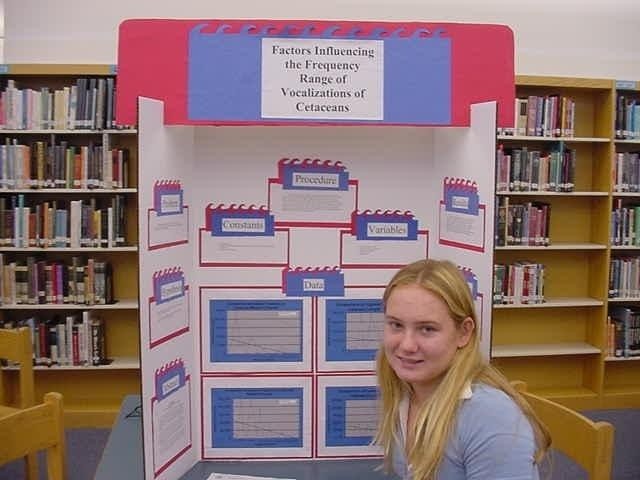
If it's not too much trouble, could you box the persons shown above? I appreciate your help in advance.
[369,259,553,480]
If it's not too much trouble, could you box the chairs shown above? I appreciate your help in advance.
[0,325,66,480]
[509,379,615,480]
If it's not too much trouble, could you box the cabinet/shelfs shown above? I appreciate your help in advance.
[0,65,141,430]
[496,74,640,414]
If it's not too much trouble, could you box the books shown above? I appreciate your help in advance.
[607,94,640,358]
[494,94,578,306]
[0,79,139,366]
